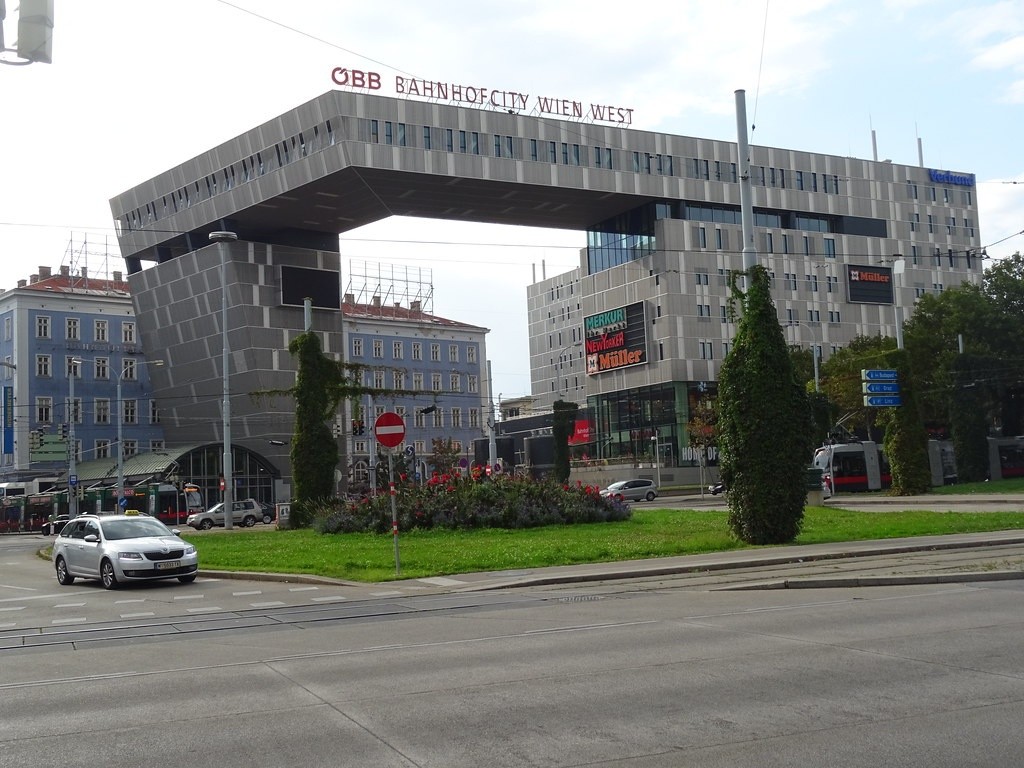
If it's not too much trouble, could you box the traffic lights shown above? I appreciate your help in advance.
[355,419,365,436]
[420,404,438,416]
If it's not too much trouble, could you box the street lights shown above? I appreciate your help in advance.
[558,340,581,398]
[72,356,163,515]
[779,323,819,390]
[651,430,660,488]
[207,230,237,533]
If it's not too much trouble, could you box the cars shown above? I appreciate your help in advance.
[599,479,658,502]
[256,500,276,524]
[53,510,198,590]
[709,480,726,495]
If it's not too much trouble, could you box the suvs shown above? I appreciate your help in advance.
[185,498,265,531]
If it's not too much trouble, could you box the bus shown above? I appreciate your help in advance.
[0,482,203,534]
[811,436,1024,493]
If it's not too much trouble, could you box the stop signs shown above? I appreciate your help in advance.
[375,412,406,448]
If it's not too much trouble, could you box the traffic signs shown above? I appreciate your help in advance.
[863,394,901,407]
[861,369,898,381]
[862,382,900,395]
